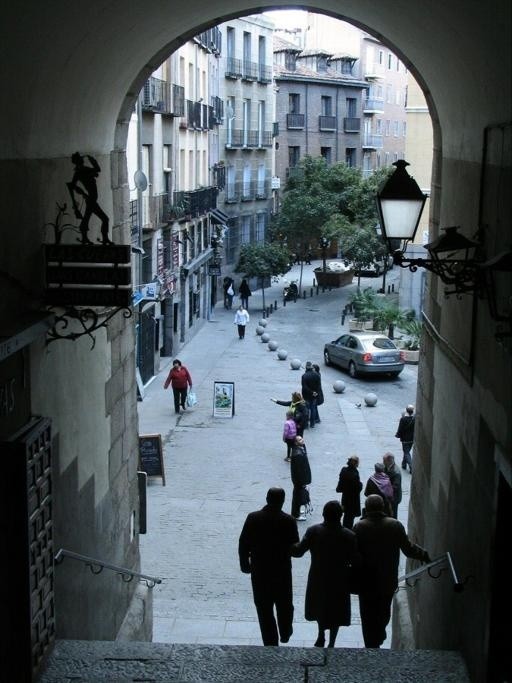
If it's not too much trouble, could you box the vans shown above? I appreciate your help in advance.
[352,252,393,276]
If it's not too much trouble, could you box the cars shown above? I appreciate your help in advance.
[324,331,406,377]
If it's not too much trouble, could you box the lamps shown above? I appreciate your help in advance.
[415,216,512,326]
[370,150,496,307]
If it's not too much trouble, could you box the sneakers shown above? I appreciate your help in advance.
[314,636,326,647]
[284,456,291,463]
[401,464,412,474]
[293,515,307,522]
[304,418,320,428]
[224,302,249,340]
[175,404,187,414]
[280,626,296,643]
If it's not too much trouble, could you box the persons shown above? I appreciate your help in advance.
[287,497,354,648]
[290,280,297,298]
[233,304,250,340]
[224,275,234,310]
[237,485,301,646]
[238,279,252,310]
[162,357,195,414]
[349,491,433,648]
[267,358,416,527]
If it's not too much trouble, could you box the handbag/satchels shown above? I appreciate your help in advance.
[227,283,235,297]
[186,387,198,407]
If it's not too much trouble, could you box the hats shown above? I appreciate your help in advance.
[291,391,302,402]
[305,361,314,370]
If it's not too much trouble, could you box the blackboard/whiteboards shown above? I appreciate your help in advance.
[138,434,165,478]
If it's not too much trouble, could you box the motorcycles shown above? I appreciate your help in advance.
[283,277,300,300]
